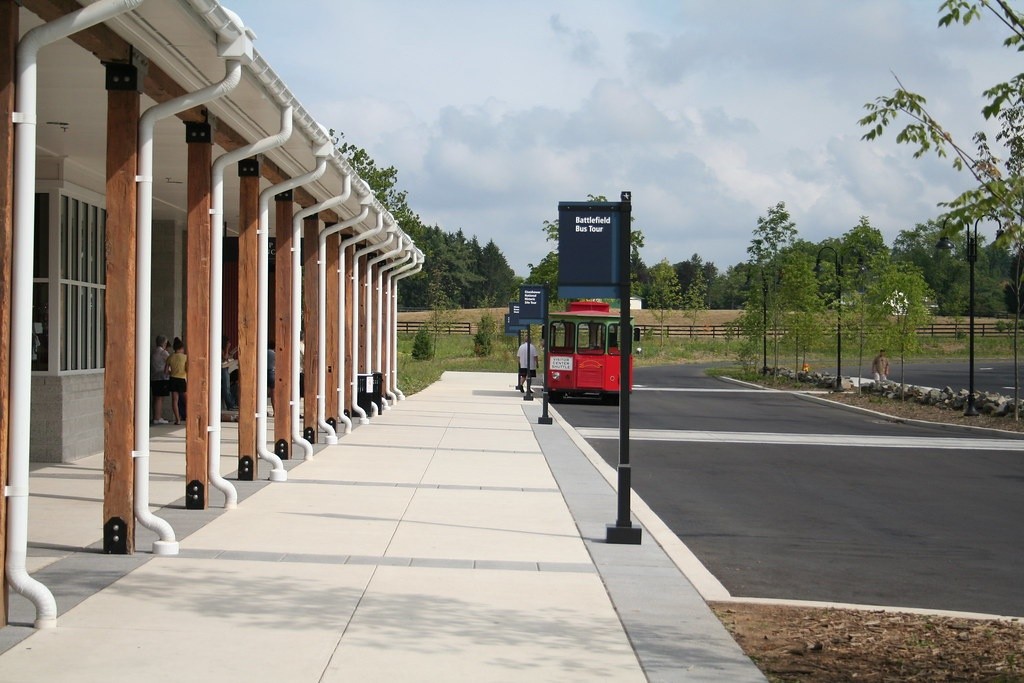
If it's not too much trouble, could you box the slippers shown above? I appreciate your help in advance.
[175,422,180,424]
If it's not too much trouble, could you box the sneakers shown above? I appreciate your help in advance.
[154,418,168,424]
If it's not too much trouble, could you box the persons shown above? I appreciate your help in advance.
[223,336,240,410]
[517,335,538,393]
[300,331,304,398]
[150,335,187,424]
[268,340,274,410]
[872,350,889,381]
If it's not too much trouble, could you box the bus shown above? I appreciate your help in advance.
[541,302,641,405]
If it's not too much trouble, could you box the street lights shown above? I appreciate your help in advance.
[936,214,1005,416]
[813,245,867,391]
[744,264,785,378]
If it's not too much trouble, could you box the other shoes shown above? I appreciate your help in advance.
[530,390,533,393]
[267,412,273,416]
[229,406,239,410]
[300,415,304,418]
[520,385,524,393]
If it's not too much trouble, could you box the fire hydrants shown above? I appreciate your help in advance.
[802,363,809,373]
